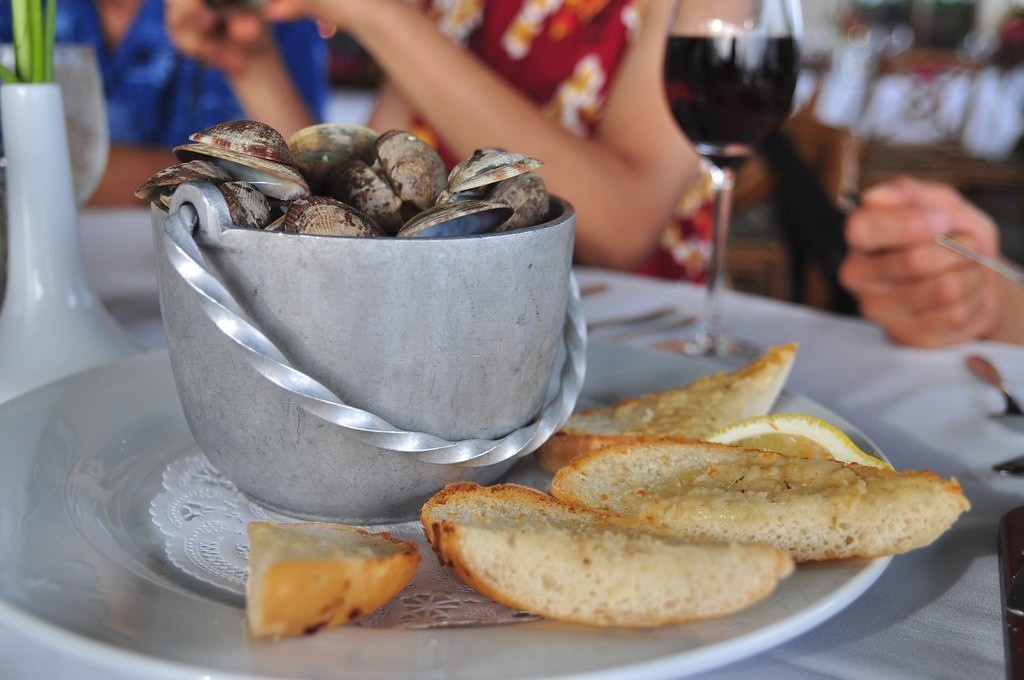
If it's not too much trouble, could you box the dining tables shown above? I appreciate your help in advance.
[1,206,1024,680]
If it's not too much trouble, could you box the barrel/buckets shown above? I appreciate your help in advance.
[146,188,591,526]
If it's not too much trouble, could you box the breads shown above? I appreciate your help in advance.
[540,342,800,476]
[421,482,795,626]
[550,439,974,564]
[244,520,422,639]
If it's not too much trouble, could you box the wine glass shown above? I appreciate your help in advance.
[663,1,805,356]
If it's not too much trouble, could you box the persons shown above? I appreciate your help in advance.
[836,176,1024,350]
[164,0,761,286]
[0,0,330,214]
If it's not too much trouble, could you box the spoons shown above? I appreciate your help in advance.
[965,356,1024,440]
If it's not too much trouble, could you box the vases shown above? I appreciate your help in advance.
[1,83,163,407]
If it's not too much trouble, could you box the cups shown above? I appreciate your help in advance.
[1,45,108,208]
[998,506,1023,680]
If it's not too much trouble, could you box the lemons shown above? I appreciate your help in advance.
[702,412,898,467]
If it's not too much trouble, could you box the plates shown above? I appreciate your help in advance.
[0,342,906,680]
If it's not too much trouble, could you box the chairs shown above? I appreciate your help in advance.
[714,121,859,317]
